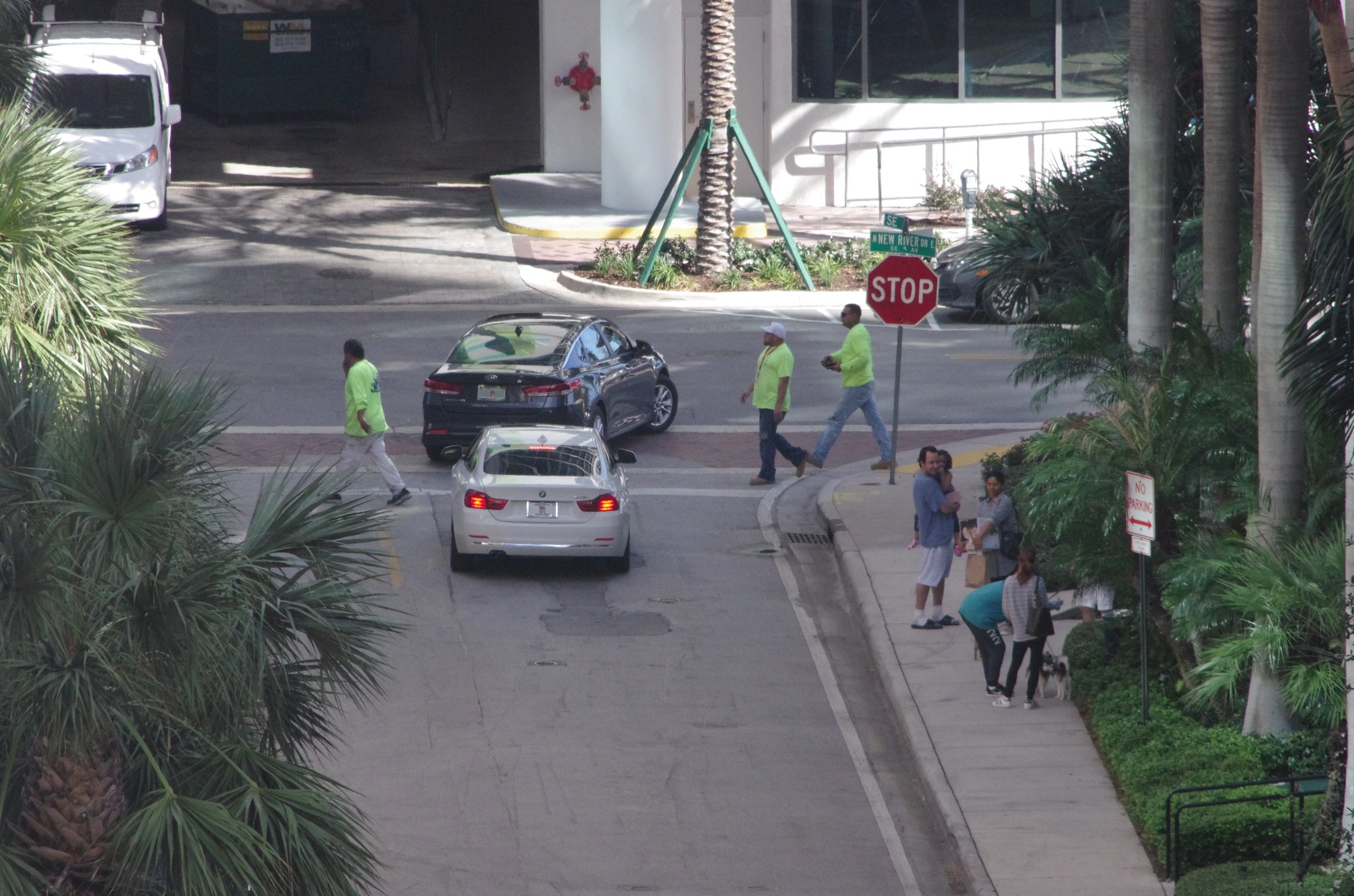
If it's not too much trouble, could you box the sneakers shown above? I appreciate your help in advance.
[908,538,919,550]
[870,459,897,470]
[319,494,343,505]
[795,450,807,476]
[986,685,1014,697]
[991,697,1012,708]
[388,489,413,506]
[952,544,961,555]
[749,475,773,485]
[806,452,823,468]
[1025,699,1039,709]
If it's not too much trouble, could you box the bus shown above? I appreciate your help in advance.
[423,312,678,459]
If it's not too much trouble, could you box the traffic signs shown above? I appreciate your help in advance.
[1126,472,1158,541]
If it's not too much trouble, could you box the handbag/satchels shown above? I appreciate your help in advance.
[964,546,995,588]
[1001,529,1036,561]
[955,518,1000,552]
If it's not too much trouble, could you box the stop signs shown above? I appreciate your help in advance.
[868,255,941,328]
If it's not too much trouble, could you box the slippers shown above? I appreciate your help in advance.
[910,618,942,629]
[934,615,959,624]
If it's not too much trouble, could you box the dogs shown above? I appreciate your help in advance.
[1026,651,1054,699]
[1048,655,1074,700]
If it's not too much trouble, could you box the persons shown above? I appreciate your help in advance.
[1068,563,1115,623]
[741,319,813,487]
[972,471,1026,639]
[802,302,899,472]
[959,578,1063,698]
[991,546,1065,710]
[960,518,977,553]
[906,450,963,558]
[317,338,413,508]
[908,446,960,631]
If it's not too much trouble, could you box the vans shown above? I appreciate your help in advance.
[23,3,183,230]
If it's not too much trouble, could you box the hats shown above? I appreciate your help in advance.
[763,321,786,338]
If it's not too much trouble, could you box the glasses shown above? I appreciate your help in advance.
[841,312,854,316]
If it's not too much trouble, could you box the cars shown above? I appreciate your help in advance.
[441,422,639,577]
